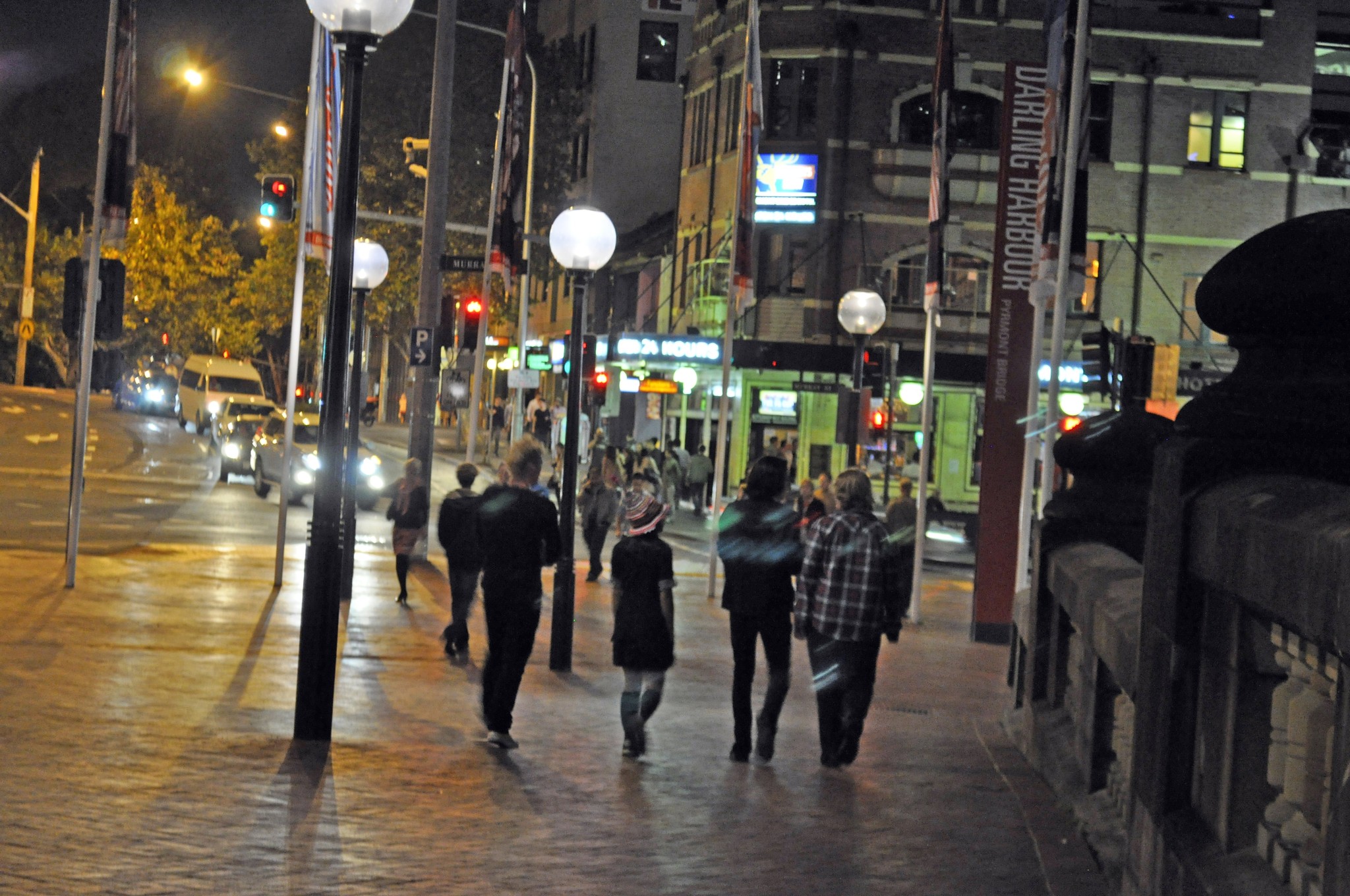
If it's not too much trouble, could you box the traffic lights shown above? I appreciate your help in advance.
[465,299,482,353]
[439,295,456,350]
[1126,340,1157,400]
[1080,330,1110,399]
[259,173,295,225]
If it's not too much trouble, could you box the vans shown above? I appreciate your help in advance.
[178,355,265,435]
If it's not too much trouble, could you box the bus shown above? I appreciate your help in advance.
[724,364,1120,518]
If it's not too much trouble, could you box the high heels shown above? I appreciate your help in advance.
[396,590,407,603]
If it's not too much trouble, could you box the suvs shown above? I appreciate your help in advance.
[249,409,384,511]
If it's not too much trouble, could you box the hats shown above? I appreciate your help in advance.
[622,489,670,537]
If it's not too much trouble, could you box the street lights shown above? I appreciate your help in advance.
[545,204,618,676]
[179,64,316,589]
[837,288,887,470]
[289,0,418,746]
[338,236,389,605]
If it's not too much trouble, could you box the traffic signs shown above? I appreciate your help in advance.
[408,327,433,368]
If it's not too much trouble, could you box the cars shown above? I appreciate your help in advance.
[111,366,178,417]
[219,414,267,485]
[208,397,286,448]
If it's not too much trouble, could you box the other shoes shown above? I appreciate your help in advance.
[757,720,776,762]
[586,565,602,582]
[622,739,639,758]
[729,750,749,762]
[439,623,469,654]
[486,730,520,748]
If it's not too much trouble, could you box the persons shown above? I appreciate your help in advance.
[810,471,835,516]
[379,457,429,604]
[478,433,564,746]
[486,394,798,581]
[713,456,807,763]
[795,465,901,771]
[882,476,917,614]
[438,463,494,663]
[792,478,826,591]
[609,486,676,758]
